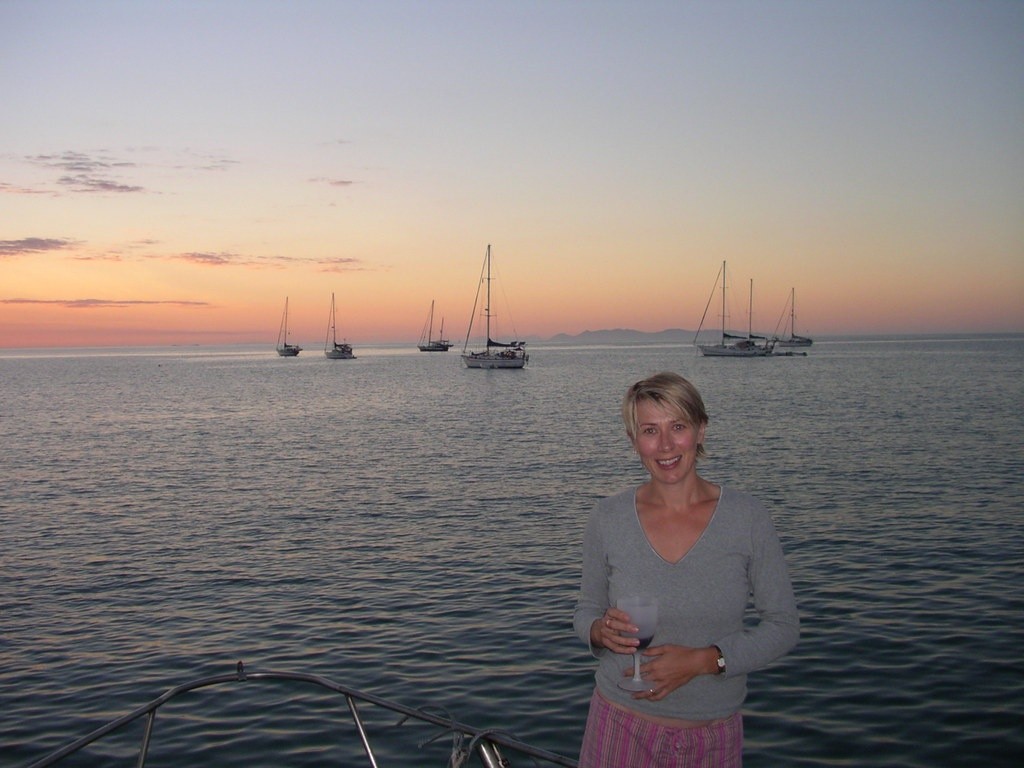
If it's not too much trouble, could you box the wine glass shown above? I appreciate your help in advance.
[617,596,658,691]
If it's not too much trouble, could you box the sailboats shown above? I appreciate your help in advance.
[692,261,767,356]
[324,292,358,359]
[416,299,449,351]
[748,277,815,347]
[433,317,454,347]
[274,296,303,356]
[461,244,529,367]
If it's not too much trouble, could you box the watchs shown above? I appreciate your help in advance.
[714,645,726,673]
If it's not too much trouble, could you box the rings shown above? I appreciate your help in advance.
[606,619,610,627]
[648,689,656,696]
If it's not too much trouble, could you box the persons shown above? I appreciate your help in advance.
[573,371,801,768]
